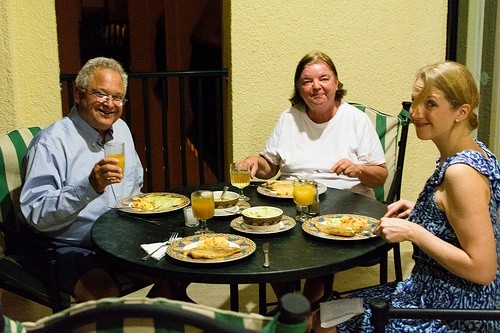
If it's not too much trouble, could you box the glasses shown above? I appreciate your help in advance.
[79,85,128,107]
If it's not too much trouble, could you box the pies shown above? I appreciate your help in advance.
[182,236,241,258]
[314,223,354,236]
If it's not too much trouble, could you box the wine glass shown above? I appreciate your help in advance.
[293,179,318,223]
[191,191,215,235]
[229,161,252,201]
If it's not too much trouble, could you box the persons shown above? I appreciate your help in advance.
[19,56,194,303]
[236,50,388,333]
[311,61,500,333]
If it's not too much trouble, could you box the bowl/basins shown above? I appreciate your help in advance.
[242,205,283,229]
[201,190,239,214]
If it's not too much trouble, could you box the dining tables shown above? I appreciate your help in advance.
[91,182,401,316]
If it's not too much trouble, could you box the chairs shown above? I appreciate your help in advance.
[0,293,312,333]
[0,126,153,314]
[350,101,412,285]
[369,297,500,333]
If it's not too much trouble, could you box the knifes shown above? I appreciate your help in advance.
[127,215,161,225]
[262,240,270,268]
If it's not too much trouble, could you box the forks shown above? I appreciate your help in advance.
[142,232,179,260]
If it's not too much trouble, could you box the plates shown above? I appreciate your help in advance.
[256,179,328,198]
[166,233,256,264]
[230,214,297,234]
[301,214,384,240]
[115,192,192,214]
[214,202,251,217]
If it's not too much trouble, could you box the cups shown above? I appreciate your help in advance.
[103,143,125,179]
[183,205,200,228]
